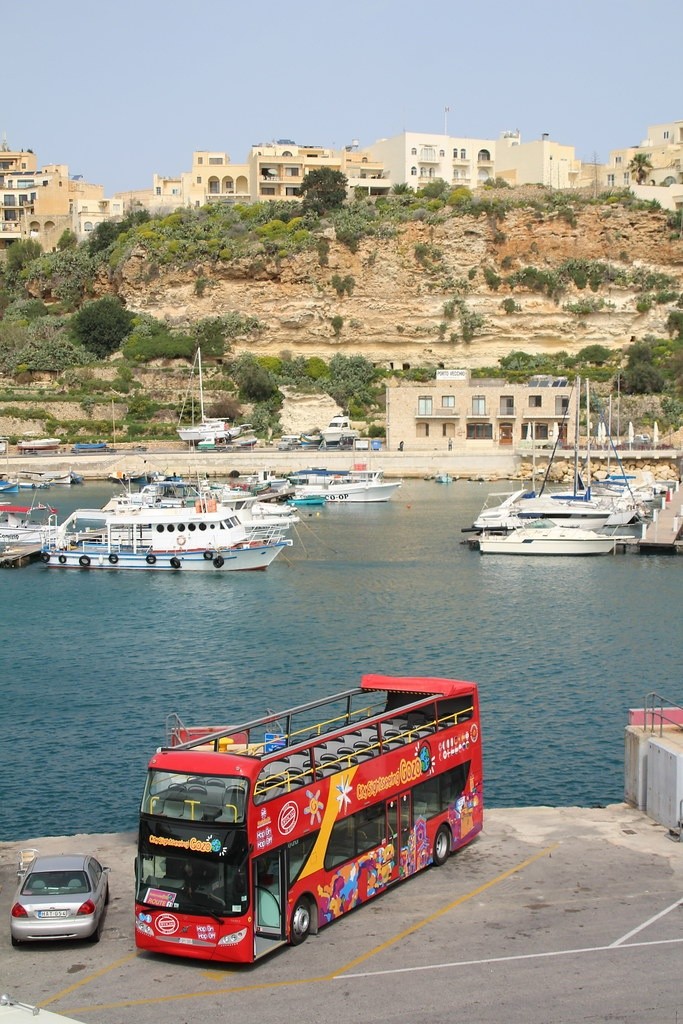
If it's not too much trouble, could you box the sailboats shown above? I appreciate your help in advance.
[175,346,242,445]
[460,374,678,557]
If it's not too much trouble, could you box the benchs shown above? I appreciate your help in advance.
[82,540,122,554]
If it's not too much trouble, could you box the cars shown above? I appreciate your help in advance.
[8,853,108,945]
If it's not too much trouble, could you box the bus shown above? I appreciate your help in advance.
[277,435,300,450]
[132,672,482,963]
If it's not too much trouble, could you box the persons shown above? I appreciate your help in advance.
[400,441,404,451]
[559,438,562,449]
[184,862,194,878]
[448,438,453,451]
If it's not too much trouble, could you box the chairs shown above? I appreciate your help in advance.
[324,803,428,869]
[159,709,324,823]
[322,762,341,777]
[320,753,339,766]
[15,848,41,882]
[32,880,45,889]
[68,879,82,886]
[325,710,472,770]
[314,742,327,762]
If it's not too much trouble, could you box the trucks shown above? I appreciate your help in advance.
[196,437,241,452]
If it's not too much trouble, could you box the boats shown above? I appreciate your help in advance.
[231,422,257,448]
[300,432,323,446]
[100,466,403,535]
[321,406,359,447]
[50,465,82,484]
[0,473,18,493]
[38,496,292,570]
[18,470,51,490]
[16,438,61,450]
[73,440,108,449]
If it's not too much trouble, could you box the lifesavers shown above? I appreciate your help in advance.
[108,554,118,564]
[145,554,156,564]
[170,558,180,568]
[59,555,66,563]
[78,556,90,566]
[40,553,50,563]
[203,551,224,568]
